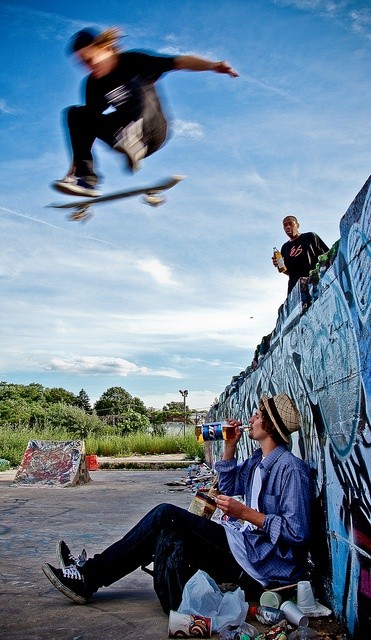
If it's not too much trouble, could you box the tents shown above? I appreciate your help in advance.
[9,439,93,488]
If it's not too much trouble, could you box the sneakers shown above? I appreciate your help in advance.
[54,176,104,198]
[111,145,140,178]
[56,540,87,569]
[41,563,89,605]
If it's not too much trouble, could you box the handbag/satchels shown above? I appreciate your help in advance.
[142,527,191,615]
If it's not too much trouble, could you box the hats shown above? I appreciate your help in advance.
[261,392,301,444]
[60,27,103,59]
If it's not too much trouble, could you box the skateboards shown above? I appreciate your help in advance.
[43,175,184,220]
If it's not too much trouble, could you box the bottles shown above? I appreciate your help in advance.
[250,606,285,625]
[272,247,286,273]
[194,421,252,442]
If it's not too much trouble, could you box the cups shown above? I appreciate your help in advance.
[168,610,213,639]
[298,581,317,611]
[258,591,282,609]
[280,601,310,627]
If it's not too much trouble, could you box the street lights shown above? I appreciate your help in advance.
[178,390,188,436]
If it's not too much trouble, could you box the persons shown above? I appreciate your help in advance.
[48,26,240,198]
[41,390,311,605]
[271,215,330,298]
